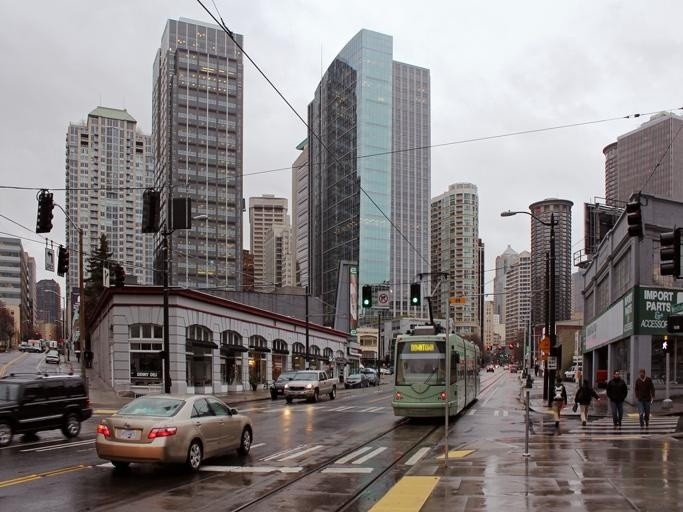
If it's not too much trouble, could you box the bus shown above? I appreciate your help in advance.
[391,323,480,420]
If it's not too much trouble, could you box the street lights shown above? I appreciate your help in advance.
[511,318,527,378]
[45,289,67,363]
[161,214,208,395]
[501,211,556,406]
[21,319,31,343]
[39,309,50,335]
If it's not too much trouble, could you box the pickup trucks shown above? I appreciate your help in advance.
[284,370,339,403]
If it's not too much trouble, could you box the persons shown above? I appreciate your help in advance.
[634,368,655,427]
[534,363,539,377]
[549,376,568,428]
[605,369,628,427]
[574,377,600,426]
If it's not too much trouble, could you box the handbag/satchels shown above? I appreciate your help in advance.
[572,403,578,412]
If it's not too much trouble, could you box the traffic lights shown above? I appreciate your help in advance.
[410,284,421,307]
[35,193,54,234]
[362,286,372,308]
[625,199,644,241]
[657,227,681,277]
[56,245,70,277]
[661,336,671,353]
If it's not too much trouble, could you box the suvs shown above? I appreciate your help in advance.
[269,371,295,400]
[0,373,92,448]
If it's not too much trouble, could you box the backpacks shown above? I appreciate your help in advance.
[553,385,564,400]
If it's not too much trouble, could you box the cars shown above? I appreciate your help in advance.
[361,368,376,374]
[344,373,368,391]
[46,350,60,365]
[360,364,364,369]
[365,373,379,387]
[376,368,390,375]
[94,393,254,473]
[484,361,520,375]
[18,344,43,353]
[564,365,583,381]
